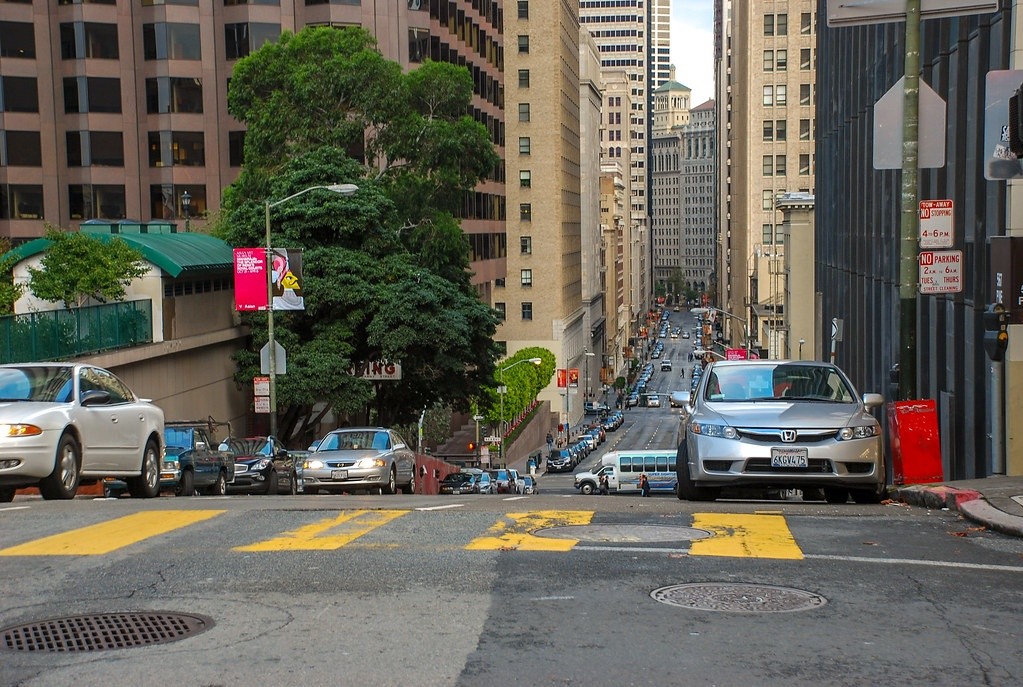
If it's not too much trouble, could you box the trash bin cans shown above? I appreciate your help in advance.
[526,458,536,474]
[636,364,640,372]
[529,450,542,469]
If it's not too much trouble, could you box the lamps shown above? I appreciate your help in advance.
[613,215,623,230]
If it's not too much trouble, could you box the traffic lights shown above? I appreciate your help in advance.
[469,442,474,450]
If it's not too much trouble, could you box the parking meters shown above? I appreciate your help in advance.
[983,302,1005,476]
[889,364,900,486]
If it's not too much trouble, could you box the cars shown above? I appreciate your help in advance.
[300,426,418,495]
[438,468,537,494]
[670,359,887,505]
[545,410,624,472]
[0,362,166,502]
[628,310,703,407]
[221,435,322,495]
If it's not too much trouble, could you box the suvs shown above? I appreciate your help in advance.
[585,401,610,414]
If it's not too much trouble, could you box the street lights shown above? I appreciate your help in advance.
[263,183,359,440]
[499,356,541,463]
[691,308,749,360]
[566,352,595,443]
[180,190,193,233]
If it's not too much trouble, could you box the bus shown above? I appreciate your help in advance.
[574,450,678,495]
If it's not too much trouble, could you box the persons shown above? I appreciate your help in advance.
[640,475,651,497]
[626,400,630,410]
[599,475,610,496]
[688,353,693,362]
[680,369,684,378]
[559,423,564,437]
[546,433,553,451]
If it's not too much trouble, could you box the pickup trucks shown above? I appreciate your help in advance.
[103,425,234,497]
